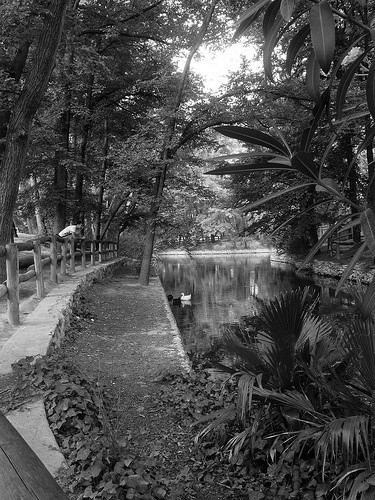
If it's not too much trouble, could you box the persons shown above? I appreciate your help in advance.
[56,220,86,262]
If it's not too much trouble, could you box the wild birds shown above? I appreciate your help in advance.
[179,293,191,300]
[167,294,173,299]
[173,297,181,303]
